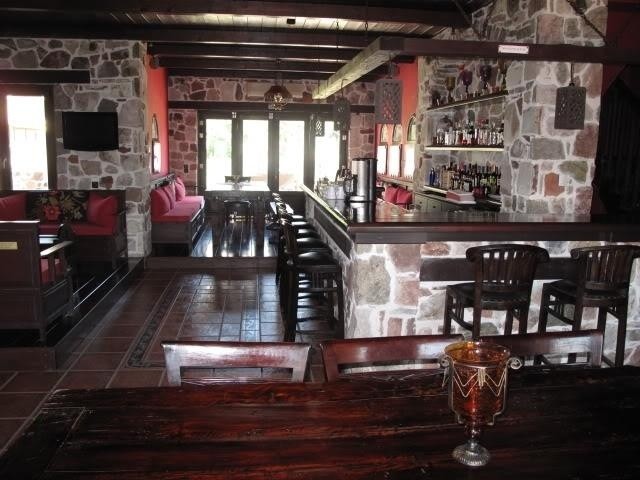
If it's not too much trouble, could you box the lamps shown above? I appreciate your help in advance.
[264,71,292,111]
[310,0,402,137]
[554,62,586,130]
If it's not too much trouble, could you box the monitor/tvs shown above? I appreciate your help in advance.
[61,112,119,151]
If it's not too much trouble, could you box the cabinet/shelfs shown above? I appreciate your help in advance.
[413,90,509,212]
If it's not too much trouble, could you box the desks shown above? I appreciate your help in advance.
[0,364,640,480]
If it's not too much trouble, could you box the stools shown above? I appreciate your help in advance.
[266,195,344,342]
[539,244,640,363]
[444,244,549,338]
[223,199,254,227]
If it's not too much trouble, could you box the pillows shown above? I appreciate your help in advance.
[151,177,186,217]
[26,190,118,227]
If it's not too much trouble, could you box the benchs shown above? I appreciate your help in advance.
[0,220,75,341]
[0,190,131,270]
[150,172,208,256]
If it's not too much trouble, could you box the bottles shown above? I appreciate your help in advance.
[429,115,504,201]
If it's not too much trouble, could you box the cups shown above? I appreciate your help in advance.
[326,185,346,201]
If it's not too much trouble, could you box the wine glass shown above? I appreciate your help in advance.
[439,340,524,469]
[445,76,456,103]
[462,71,472,99]
[479,64,492,96]
